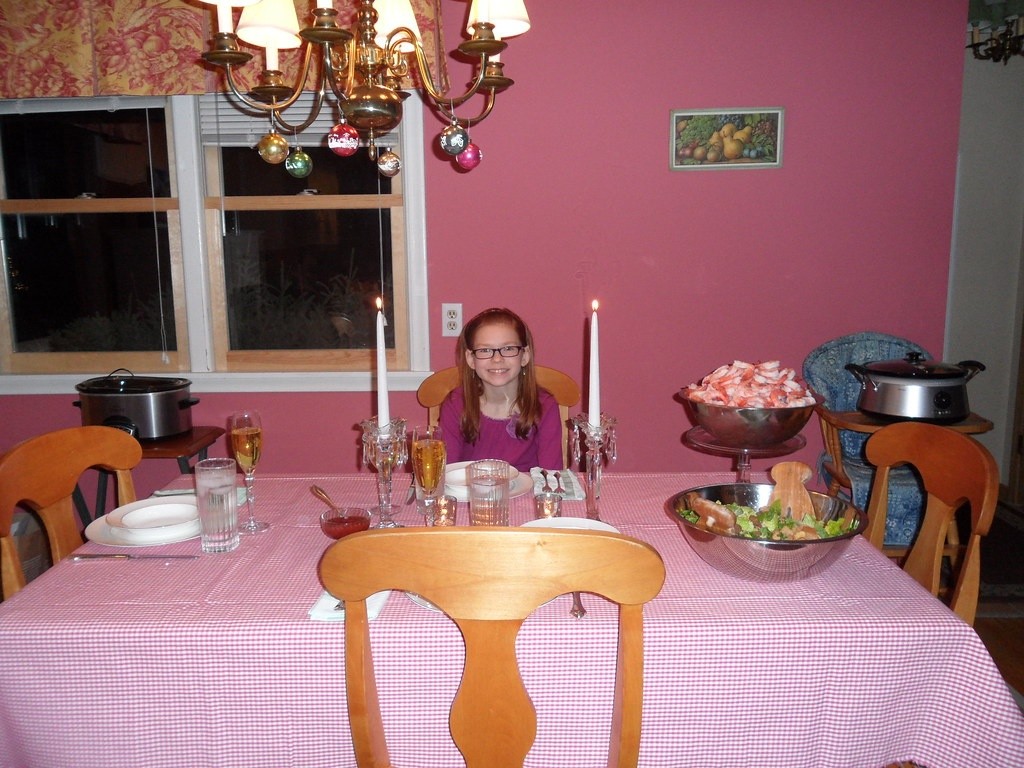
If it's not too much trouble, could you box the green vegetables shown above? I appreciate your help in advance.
[681,500,854,539]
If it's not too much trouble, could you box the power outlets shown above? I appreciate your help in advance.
[441,303,462,337]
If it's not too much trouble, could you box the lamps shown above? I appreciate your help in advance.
[200,0,531,180]
[966,17,1024,66]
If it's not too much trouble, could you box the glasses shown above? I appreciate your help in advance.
[473,346,523,359]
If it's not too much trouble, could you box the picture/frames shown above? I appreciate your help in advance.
[670,107,785,170]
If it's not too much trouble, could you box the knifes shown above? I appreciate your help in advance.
[68,553,202,559]
[406,473,415,504]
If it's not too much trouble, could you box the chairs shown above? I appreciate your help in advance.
[315,525,665,768]
[415,363,579,472]
[805,332,995,578]
[0,426,143,602]
[862,422,1001,624]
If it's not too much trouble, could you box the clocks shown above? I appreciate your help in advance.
[376,297,391,427]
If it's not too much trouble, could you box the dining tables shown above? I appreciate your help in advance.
[0,471,1023,768]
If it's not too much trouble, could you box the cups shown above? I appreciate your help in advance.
[469,459,510,527]
[534,494,562,519]
[430,494,458,527]
[194,457,240,554]
[415,472,445,515]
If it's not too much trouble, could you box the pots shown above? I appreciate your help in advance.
[844,351,987,427]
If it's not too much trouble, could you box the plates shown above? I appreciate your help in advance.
[438,460,534,503]
[405,591,559,615]
[518,516,621,535]
[84,493,201,548]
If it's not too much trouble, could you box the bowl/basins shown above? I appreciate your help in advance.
[668,482,870,584]
[320,507,372,540]
[444,463,519,495]
[679,389,826,450]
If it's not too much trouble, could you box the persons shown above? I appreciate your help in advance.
[434,308,564,472]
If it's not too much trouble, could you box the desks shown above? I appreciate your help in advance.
[832,408,994,435]
[87,427,225,520]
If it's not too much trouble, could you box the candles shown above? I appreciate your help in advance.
[589,299,601,425]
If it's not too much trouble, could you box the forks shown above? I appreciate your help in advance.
[554,472,566,493]
[540,470,553,493]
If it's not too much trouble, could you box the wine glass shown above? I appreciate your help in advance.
[412,425,446,527]
[227,409,270,535]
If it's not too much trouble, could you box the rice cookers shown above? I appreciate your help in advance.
[73,376,201,442]
